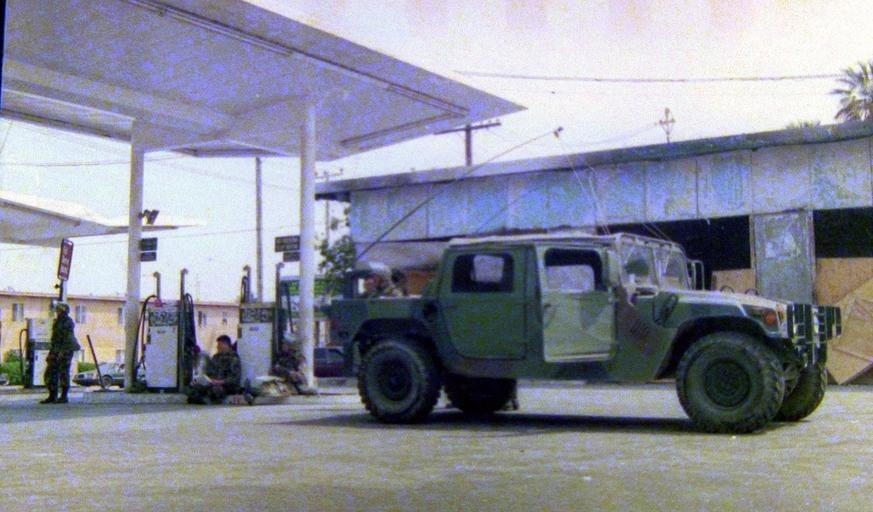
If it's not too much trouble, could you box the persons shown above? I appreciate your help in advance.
[274,333,318,395]
[39,303,80,404]
[363,270,407,298]
[187,335,242,404]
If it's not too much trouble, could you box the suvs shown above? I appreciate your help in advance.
[328,229,845,437]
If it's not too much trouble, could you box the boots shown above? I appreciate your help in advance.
[40,387,69,404]
